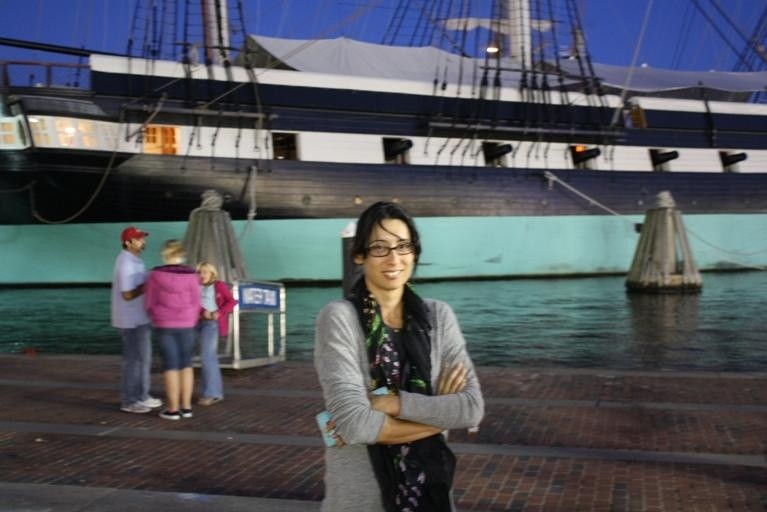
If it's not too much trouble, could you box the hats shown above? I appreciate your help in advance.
[122,227,149,241]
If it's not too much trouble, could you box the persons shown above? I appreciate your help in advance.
[110,228,163,414]
[143,239,200,420]
[315,202,485,512]
[195,260,239,406]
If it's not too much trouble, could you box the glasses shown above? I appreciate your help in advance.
[366,243,416,258]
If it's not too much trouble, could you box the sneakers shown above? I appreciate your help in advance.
[181,408,193,418]
[120,400,153,414]
[159,410,181,420]
[197,396,221,407]
[144,398,163,408]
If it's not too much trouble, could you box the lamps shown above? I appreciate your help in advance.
[486,31,500,53]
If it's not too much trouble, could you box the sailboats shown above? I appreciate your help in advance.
[0,1,765,295]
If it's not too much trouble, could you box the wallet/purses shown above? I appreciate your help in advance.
[313,387,392,448]
